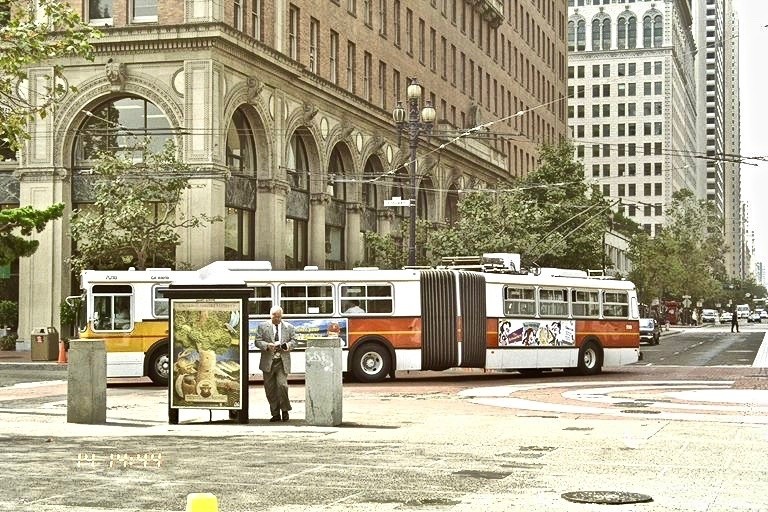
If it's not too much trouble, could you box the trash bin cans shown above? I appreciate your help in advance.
[30,326,59,361]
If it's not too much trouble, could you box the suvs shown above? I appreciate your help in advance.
[639,318,661,346]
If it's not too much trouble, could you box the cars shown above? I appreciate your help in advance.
[701,304,768,323]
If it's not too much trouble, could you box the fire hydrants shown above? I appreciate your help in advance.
[665,321,671,331]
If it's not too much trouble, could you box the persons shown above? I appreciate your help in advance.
[255,305,298,422]
[344,300,365,313]
[689,308,698,326]
[731,311,739,332]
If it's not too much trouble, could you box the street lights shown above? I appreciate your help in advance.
[393,77,437,267]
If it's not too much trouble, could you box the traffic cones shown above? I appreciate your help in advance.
[55,340,68,364]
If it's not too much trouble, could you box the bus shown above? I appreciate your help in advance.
[65,198,640,383]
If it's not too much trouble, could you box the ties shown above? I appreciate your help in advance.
[274,324,279,341]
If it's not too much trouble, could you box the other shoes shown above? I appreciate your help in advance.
[270,416,281,422]
[282,411,289,420]
